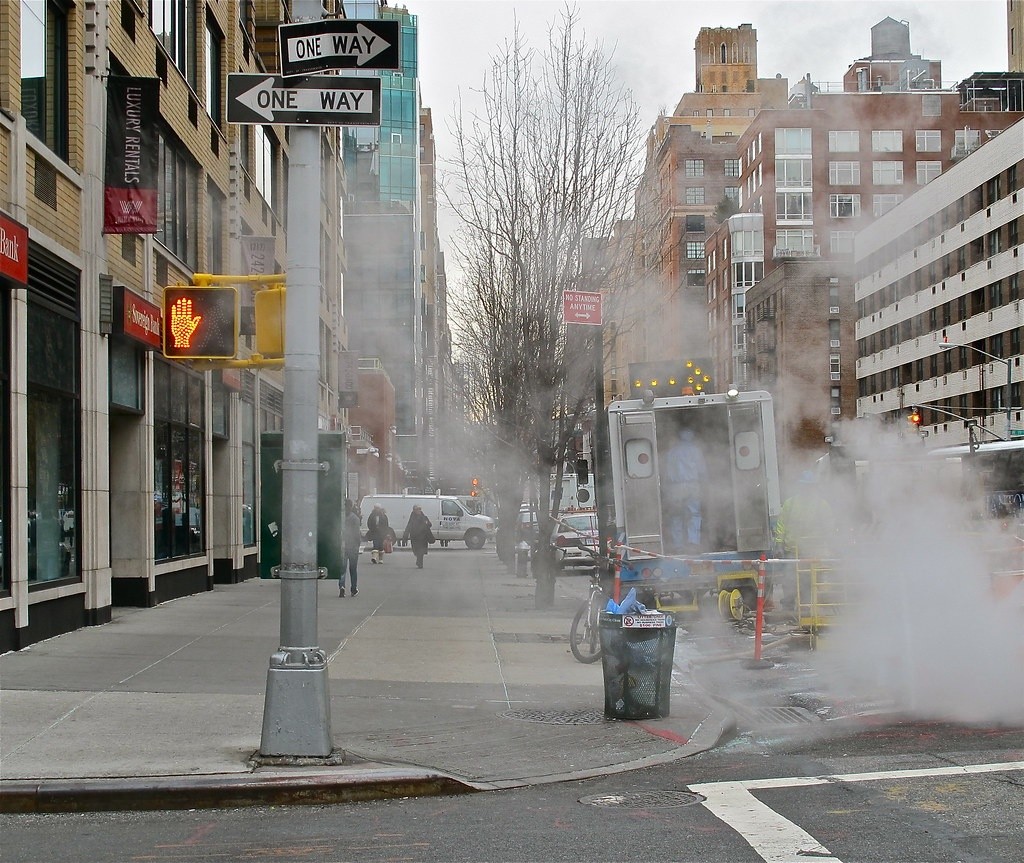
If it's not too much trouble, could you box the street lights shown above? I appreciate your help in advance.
[937,340,1012,442]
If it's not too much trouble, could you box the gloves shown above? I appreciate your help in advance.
[402,539,408,546]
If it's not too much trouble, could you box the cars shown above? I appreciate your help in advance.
[517,499,539,559]
[549,512,600,575]
[0,509,75,583]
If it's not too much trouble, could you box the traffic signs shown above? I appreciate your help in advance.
[277,18,401,79]
[562,290,602,326]
[227,72,383,127]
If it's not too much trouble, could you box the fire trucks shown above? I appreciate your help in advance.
[578,356,784,609]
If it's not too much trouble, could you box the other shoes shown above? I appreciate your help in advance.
[339,586,345,597]
[416,561,422,568]
[352,589,359,597]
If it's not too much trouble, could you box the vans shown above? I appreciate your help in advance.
[549,472,595,514]
[359,488,496,550]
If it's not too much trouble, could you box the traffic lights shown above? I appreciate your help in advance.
[908,414,920,430]
[162,286,239,360]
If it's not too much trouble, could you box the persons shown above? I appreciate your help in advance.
[440,540,448,547]
[354,500,364,553]
[367,504,388,564]
[397,505,432,569]
[339,499,359,598]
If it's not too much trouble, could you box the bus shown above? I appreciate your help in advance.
[853,440,1024,602]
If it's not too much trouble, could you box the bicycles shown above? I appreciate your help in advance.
[534,541,566,606]
[570,539,636,665]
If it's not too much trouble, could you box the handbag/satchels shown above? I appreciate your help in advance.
[427,528,435,543]
[384,541,393,553]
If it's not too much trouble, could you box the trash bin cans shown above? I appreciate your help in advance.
[599,609,677,721]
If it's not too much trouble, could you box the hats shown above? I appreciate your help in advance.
[374,503,381,508]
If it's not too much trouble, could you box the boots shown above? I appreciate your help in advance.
[379,550,384,564]
[372,550,378,564]
